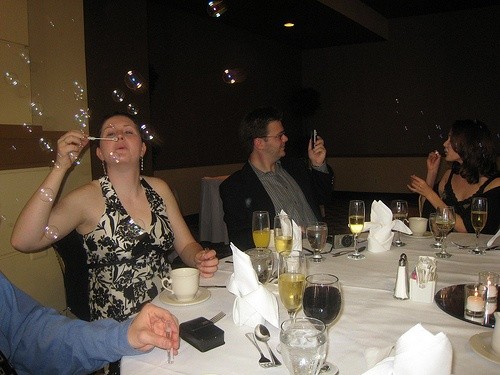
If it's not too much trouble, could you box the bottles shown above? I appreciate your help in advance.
[394,252,408,299]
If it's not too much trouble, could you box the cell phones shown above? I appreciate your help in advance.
[311,129,318,149]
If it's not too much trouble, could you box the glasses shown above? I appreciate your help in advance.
[258,132,285,141]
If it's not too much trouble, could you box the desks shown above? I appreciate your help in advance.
[200,176,231,247]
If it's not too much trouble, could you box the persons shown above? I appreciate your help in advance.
[11,115,219,322]
[0,270,180,375]
[220,109,334,252]
[407,121,500,235]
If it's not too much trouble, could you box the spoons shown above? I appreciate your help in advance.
[254,324,282,367]
[331,246,366,256]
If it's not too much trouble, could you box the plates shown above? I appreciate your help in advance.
[159,287,211,306]
[434,283,500,328]
[402,232,433,238]
[469,332,500,365]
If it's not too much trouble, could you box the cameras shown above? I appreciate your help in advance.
[333,234,355,249]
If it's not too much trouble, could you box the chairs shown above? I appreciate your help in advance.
[52,230,92,322]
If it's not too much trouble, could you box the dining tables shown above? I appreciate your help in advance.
[119,231,500,375]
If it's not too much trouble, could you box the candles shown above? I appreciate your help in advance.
[466,280,497,312]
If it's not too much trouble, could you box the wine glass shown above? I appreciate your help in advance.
[300,274,344,375]
[434,205,456,258]
[274,215,293,273]
[389,200,409,247]
[276,251,306,354]
[468,197,487,255]
[252,211,271,256]
[305,222,328,262]
[429,213,447,249]
[347,200,366,260]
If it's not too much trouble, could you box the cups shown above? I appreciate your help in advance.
[279,318,328,375]
[245,248,275,286]
[161,268,200,302]
[404,217,428,237]
[463,271,500,320]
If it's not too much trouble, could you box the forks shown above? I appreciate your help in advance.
[209,311,226,323]
[245,332,274,368]
[451,241,500,250]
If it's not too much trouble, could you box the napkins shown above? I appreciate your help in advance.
[278,209,303,251]
[367,200,413,253]
[228,241,281,330]
[362,323,454,375]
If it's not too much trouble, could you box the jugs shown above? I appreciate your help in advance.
[492,310,500,354]
